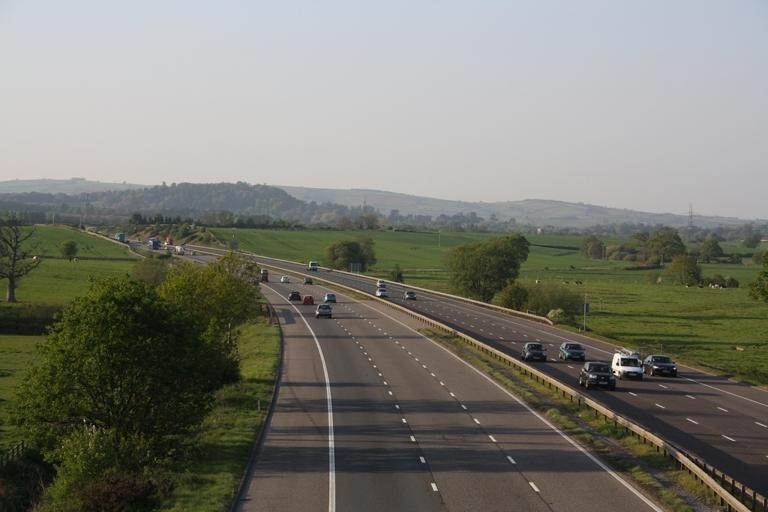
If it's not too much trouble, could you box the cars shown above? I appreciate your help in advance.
[303,276,312,285]
[325,293,337,303]
[521,341,548,362]
[281,276,289,283]
[558,341,585,362]
[315,303,332,319]
[375,279,416,302]
[642,354,677,377]
[288,291,301,301]
[303,295,315,305]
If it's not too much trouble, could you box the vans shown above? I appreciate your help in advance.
[611,353,643,381]
[309,261,318,271]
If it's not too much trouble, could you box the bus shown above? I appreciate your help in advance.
[259,269,269,282]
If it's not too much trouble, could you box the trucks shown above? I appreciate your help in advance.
[88,227,185,256]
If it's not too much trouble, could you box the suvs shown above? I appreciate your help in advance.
[579,361,616,391]
